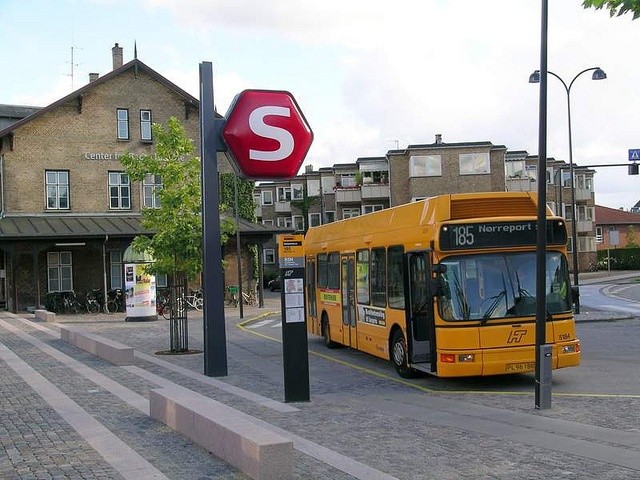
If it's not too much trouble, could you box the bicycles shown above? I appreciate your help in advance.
[177,291,202,309]
[242,289,259,308]
[85,290,100,313]
[103,289,124,312]
[227,287,238,308]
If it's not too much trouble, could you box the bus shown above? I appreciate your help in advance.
[305,190,580,377]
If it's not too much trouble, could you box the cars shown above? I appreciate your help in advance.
[268,276,281,291]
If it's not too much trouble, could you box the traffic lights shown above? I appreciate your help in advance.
[628,164,639,174]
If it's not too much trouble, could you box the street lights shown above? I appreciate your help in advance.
[528,66,606,314]
